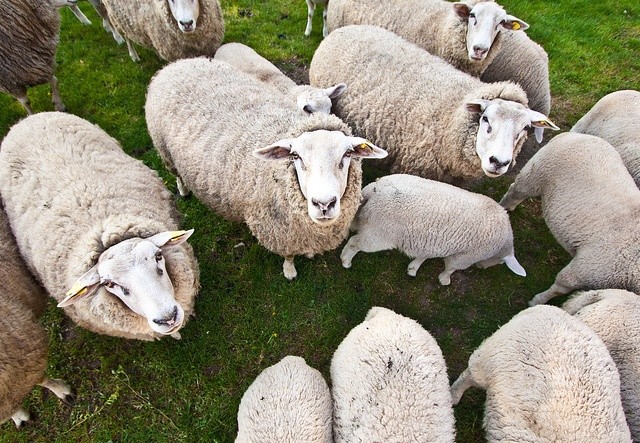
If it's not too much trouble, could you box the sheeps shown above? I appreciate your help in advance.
[480,28,552,143]
[340,173,527,285]
[304,0,329,39]
[66,0,125,44]
[329,304,458,442]
[449,303,634,442]
[142,54,389,281]
[0,195,77,431]
[570,88,640,189]
[0,110,202,342]
[308,24,561,187]
[0,0,79,117]
[561,287,640,442]
[325,0,531,79]
[498,131,640,306]
[233,354,334,442]
[101,0,225,63]
[213,41,348,119]
